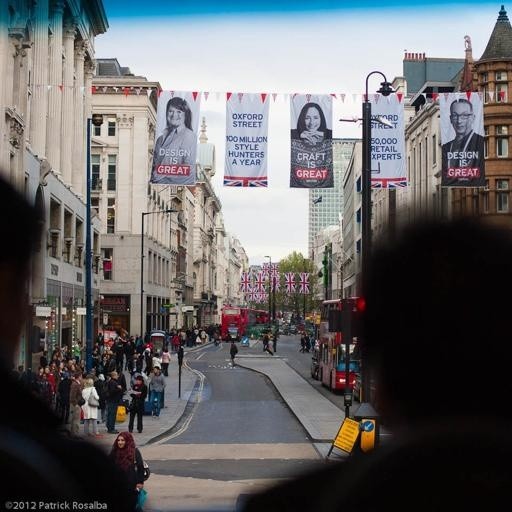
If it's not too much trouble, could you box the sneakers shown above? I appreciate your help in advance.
[83,433,91,436]
[108,430,119,434]
[93,432,102,435]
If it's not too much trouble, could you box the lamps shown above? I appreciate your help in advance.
[44,228,101,273]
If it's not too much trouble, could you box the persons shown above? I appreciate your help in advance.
[239,192,510,512]
[442,100,486,186]
[0,171,136,510]
[10,324,319,437]
[150,97,196,183]
[291,103,334,189]
[107,431,145,510]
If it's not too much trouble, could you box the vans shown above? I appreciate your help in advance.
[97,330,117,347]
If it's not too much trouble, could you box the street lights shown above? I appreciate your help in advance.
[318,266,328,300]
[141,208,179,342]
[87,113,105,373]
[362,71,395,277]
[265,256,272,315]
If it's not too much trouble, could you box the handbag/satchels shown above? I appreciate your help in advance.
[135,460,150,481]
[88,395,99,406]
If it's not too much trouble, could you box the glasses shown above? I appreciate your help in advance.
[451,110,473,120]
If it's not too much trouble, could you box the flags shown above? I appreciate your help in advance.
[241,263,311,304]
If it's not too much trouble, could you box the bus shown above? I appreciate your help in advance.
[219,304,254,342]
[316,296,364,393]
[256,309,268,324]
